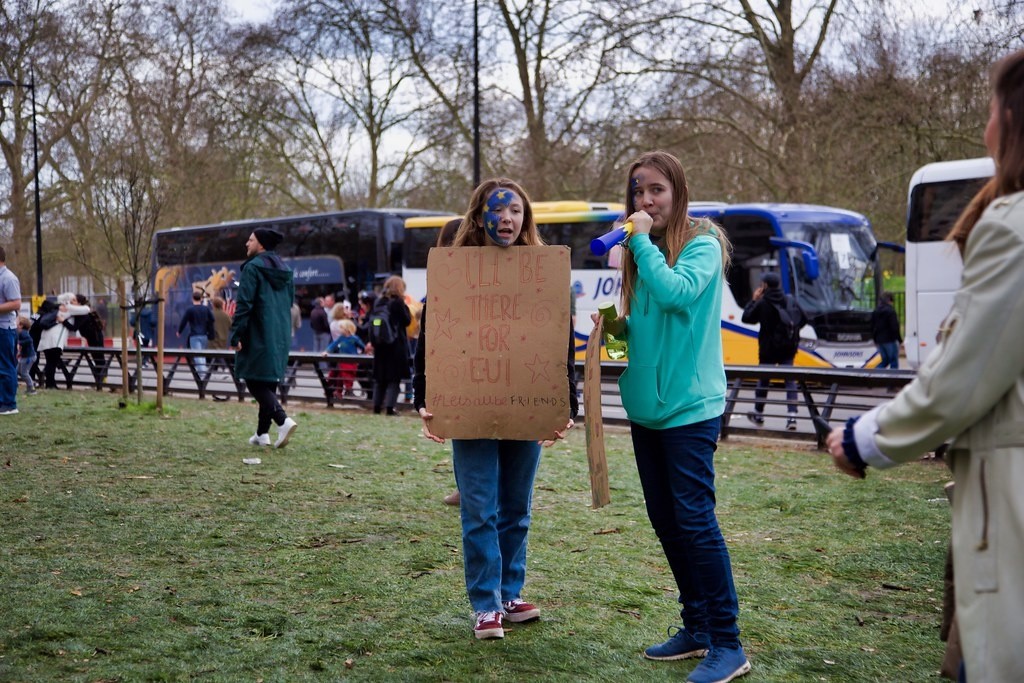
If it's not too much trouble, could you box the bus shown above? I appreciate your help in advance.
[902,157,1000,367]
[151,209,463,370]
[401,197,904,385]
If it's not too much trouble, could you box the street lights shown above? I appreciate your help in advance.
[0,70,44,302]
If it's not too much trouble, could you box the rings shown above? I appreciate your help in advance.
[828,449,832,453]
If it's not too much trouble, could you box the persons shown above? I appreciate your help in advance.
[870,291,902,394]
[130,275,576,416]
[15,292,107,396]
[826,43,1024,683]
[0,246,21,415]
[742,273,808,431]
[229,228,298,448]
[413,176,578,639]
[589,152,752,683]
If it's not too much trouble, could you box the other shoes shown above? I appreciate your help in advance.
[786,419,796,430]
[444,491,460,505]
[345,392,356,397]
[333,391,342,398]
[25,390,37,394]
[747,411,764,427]
[0,408,19,415]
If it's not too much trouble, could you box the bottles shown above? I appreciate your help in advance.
[598,302,628,360]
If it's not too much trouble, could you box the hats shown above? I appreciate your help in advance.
[254,228,285,251]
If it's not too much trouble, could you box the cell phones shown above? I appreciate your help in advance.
[811,415,866,481]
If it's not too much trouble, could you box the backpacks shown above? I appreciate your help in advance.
[40,308,58,330]
[370,298,400,346]
[771,294,801,351]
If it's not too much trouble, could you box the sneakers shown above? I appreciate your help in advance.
[274,417,297,448]
[500,599,540,623]
[249,432,270,445]
[644,626,710,661]
[474,612,504,639]
[686,644,751,683]
